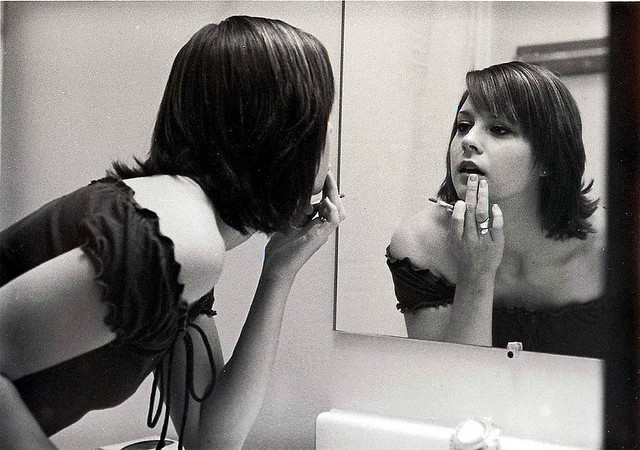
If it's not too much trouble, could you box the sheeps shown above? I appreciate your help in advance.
[451,418,501,449]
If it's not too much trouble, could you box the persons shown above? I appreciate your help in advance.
[0,14,348,450]
[385,60,608,362]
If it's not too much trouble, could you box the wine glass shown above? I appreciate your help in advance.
[320,217,327,223]
[478,229,488,235]
[477,218,489,229]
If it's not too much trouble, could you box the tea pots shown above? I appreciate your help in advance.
[332,1,607,359]
